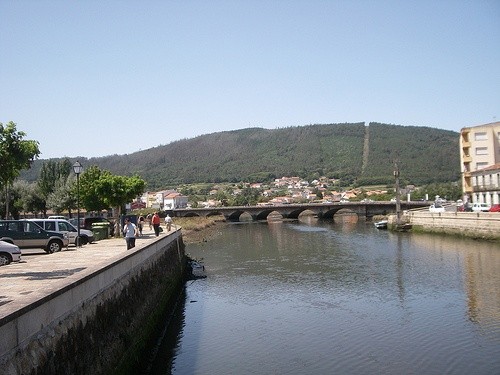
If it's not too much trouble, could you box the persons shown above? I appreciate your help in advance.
[165,214,172,231]
[123,218,137,250]
[152,211,160,236]
[146,213,152,231]
[98,210,103,218]
[137,213,145,238]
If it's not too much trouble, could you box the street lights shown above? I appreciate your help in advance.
[73,160,83,248]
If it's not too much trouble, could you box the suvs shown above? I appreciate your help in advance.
[17,218,95,247]
[0,219,70,253]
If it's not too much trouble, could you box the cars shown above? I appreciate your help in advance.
[0,240,22,266]
[46,215,116,238]
[428,197,500,213]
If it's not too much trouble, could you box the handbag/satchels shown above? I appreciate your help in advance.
[159,227,163,232]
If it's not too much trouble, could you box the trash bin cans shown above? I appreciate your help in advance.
[121,214,137,237]
[92,222,110,238]
[92,226,106,239]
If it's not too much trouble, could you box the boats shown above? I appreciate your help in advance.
[374,219,388,230]
